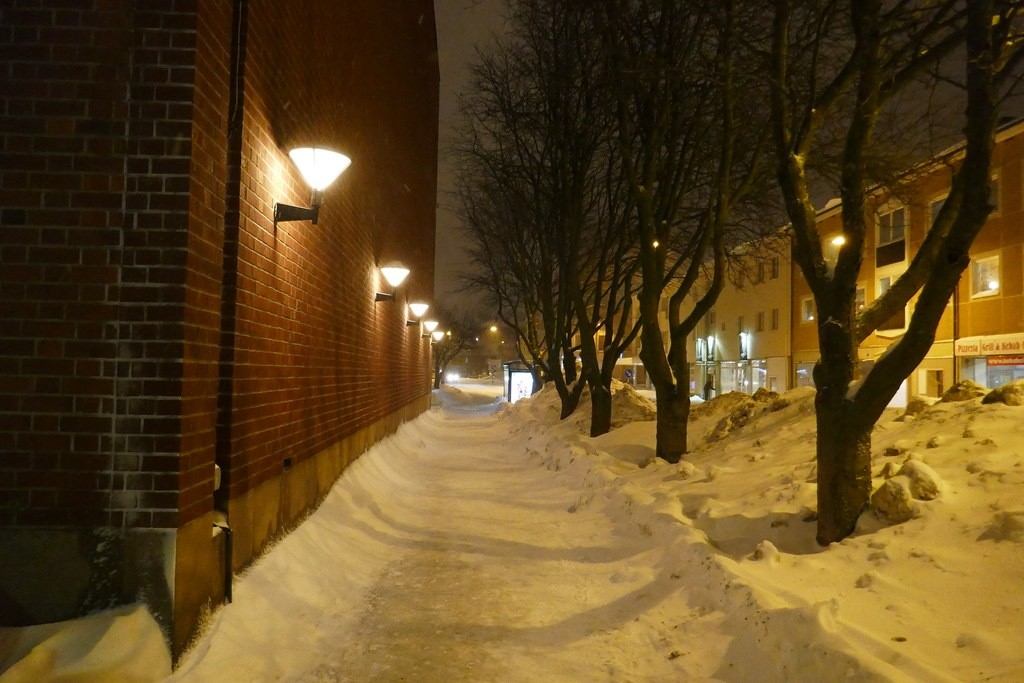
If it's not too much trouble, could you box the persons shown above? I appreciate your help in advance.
[702,378,715,401]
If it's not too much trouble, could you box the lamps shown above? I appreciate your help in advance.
[431,332,444,344]
[421,321,438,338]
[406,300,430,326]
[273,146,352,226]
[376,260,410,303]
